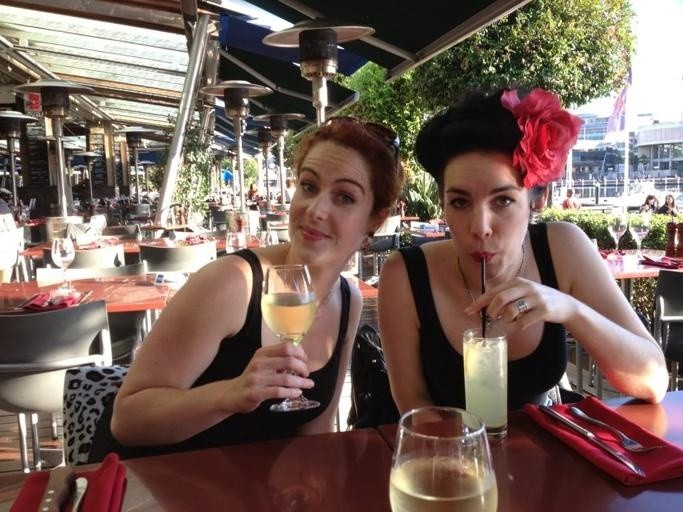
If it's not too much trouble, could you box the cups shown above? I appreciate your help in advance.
[389,407,498,512]
[461,328,508,442]
[258,231,279,247]
[225,232,248,254]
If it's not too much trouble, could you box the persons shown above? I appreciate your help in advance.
[562,188,581,211]
[639,194,660,214]
[657,194,681,217]
[100,116,405,463]
[377,86,670,427]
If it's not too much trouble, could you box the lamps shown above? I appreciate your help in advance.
[0,69,97,216]
[198,80,306,249]
[263,23,380,128]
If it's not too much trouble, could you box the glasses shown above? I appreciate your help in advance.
[323,116,401,176]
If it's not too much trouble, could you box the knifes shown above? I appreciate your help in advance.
[536,404,648,479]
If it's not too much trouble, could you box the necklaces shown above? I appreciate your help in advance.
[456,244,527,333]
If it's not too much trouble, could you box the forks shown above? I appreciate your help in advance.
[568,406,667,454]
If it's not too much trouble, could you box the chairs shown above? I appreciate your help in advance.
[363,215,405,279]
[651,269,683,390]
[0,205,290,472]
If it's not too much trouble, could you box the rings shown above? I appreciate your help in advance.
[515,300,527,311]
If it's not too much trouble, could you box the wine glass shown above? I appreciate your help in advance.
[628,215,650,262]
[260,264,320,411]
[606,217,628,256]
[51,237,76,294]
